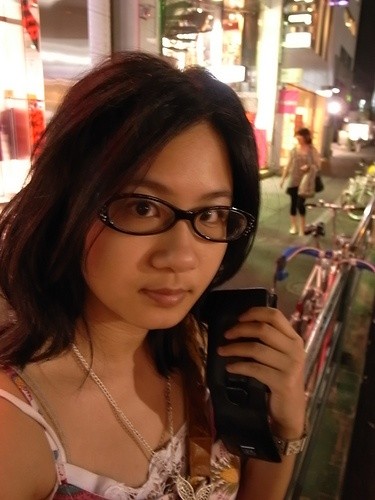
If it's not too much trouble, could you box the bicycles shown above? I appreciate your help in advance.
[272,162,375,332]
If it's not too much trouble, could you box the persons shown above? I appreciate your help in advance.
[280,128,324,236]
[0,56,307,500]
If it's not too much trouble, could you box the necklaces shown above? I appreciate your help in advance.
[70,342,195,500]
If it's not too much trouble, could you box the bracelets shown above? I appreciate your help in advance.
[275,435,308,456]
